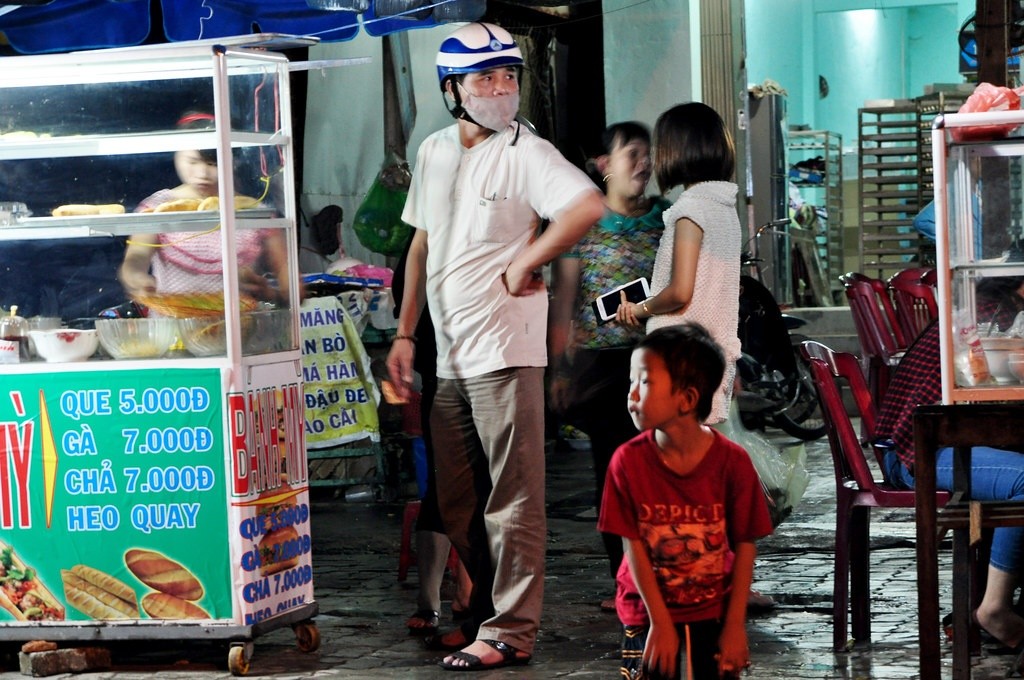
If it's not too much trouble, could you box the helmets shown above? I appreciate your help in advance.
[436,22,525,93]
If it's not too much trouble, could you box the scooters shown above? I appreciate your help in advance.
[737,274,842,441]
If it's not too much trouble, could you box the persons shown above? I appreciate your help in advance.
[385,23,745,670]
[595,322,775,680]
[116,108,305,347]
[872,237,1024,650]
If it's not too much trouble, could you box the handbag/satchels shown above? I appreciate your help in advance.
[710,389,811,532]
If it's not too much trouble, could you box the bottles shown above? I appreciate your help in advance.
[979,323,999,332]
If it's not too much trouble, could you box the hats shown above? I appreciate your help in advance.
[913,196,935,242]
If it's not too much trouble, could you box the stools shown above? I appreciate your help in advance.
[397,499,459,584]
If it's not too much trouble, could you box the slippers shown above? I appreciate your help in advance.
[453,605,469,617]
[407,609,441,633]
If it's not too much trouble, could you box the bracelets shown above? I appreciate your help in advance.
[642,303,650,315]
[391,335,418,342]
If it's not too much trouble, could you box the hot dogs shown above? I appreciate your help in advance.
[0,541,65,623]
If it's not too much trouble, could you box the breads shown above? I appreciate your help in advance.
[62,549,212,621]
[53,204,125,217]
[139,195,259,212]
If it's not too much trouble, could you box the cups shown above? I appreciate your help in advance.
[29,317,61,355]
[0,202,19,226]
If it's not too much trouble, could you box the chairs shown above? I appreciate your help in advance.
[800,266,1024,680]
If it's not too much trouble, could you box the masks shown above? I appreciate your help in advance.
[457,82,520,133]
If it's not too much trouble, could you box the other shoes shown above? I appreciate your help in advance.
[747,589,773,606]
[970,608,1024,651]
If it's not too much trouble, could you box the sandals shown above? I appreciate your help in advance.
[600,594,618,615]
[437,638,532,670]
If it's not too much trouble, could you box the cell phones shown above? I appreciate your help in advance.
[595,277,650,321]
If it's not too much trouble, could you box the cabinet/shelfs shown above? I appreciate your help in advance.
[0,44,303,369]
[932,111,1024,403]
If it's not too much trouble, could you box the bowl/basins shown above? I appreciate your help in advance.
[28,329,99,361]
[95,319,177,359]
[979,338,1024,384]
[177,316,253,357]
[245,310,291,353]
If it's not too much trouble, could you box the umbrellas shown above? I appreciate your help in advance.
[0,0,487,54]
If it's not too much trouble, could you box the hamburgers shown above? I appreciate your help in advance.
[254,482,301,575]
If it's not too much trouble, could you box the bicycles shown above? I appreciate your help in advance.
[740,217,818,426]
